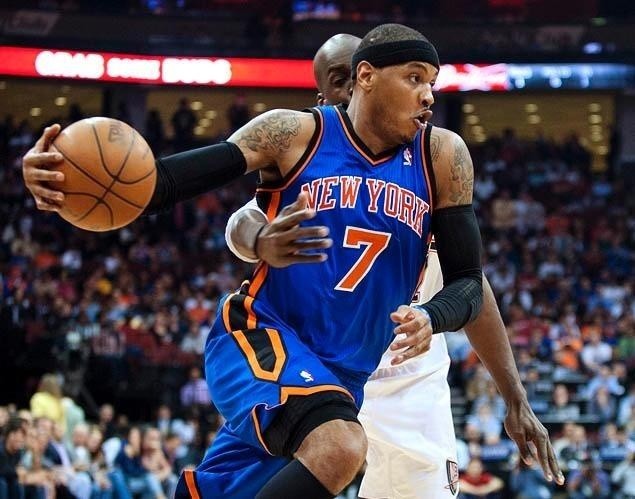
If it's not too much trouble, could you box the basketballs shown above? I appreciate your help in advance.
[46,116,157,232]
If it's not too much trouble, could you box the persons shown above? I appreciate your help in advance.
[2,24,634,498]
